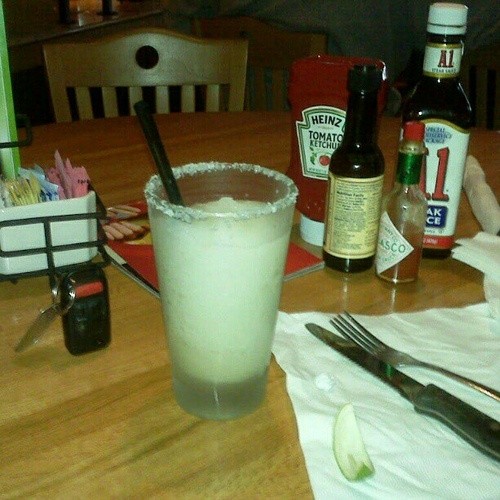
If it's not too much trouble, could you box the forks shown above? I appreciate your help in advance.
[330,310,500,402]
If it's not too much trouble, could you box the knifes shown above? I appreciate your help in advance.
[305,323,500,460]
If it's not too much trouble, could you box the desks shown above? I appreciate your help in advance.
[2,109,500,500]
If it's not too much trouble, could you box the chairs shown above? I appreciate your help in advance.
[194,22,328,113]
[42,25,251,122]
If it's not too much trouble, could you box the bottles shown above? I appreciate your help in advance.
[323,64,385,273]
[400,2,472,260]
[374,120,427,284]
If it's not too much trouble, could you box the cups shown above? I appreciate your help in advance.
[145,162,299,422]
[286,55,389,247]
